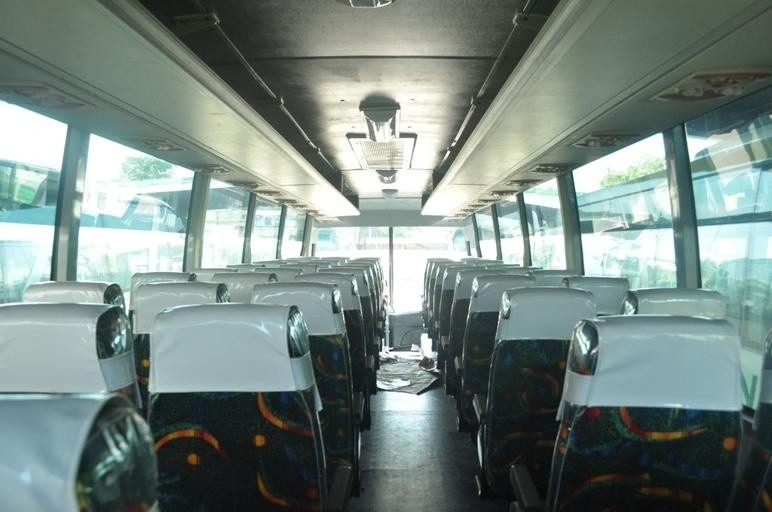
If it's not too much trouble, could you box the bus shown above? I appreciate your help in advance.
[0,158,186,316]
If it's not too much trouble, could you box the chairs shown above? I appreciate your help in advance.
[423,258,771,511]
[0,256,386,511]
